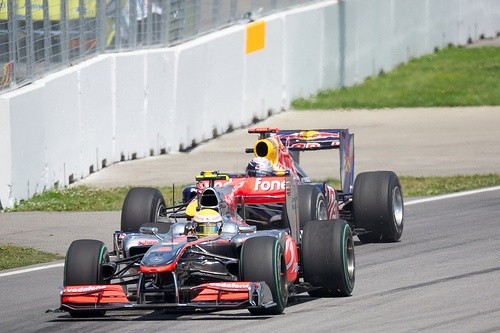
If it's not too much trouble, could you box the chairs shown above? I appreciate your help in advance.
[168,187,242,234]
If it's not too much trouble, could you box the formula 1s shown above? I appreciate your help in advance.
[43,171,356,316]
[120,128,406,242]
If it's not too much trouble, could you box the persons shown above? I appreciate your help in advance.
[245,156,273,178]
[191,208,223,239]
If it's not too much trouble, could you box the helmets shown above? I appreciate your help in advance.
[190,208,224,239]
[246,157,273,177]
